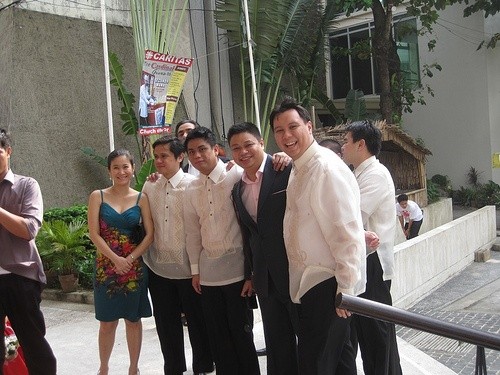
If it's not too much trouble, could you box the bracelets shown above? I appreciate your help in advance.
[129,254,135,260]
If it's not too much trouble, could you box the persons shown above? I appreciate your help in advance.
[88,148,154,375]
[270,103,366,375]
[145,118,235,184]
[0,128,57,375]
[139,75,152,126]
[395,194,423,239]
[342,121,402,375]
[183,128,294,375]
[141,136,214,375]
[227,122,380,375]
